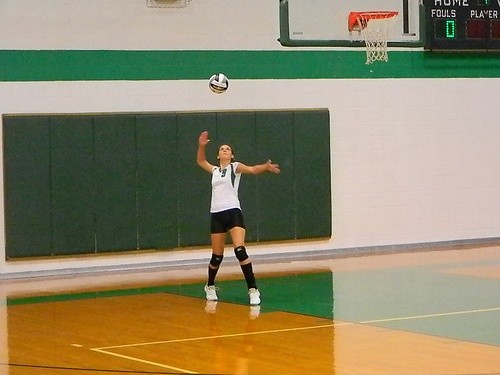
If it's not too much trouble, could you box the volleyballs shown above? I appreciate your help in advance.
[209,73,228,94]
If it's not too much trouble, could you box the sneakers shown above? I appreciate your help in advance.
[248,288,261,305]
[204,281,219,301]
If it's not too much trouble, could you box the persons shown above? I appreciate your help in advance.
[197,131,280,305]
